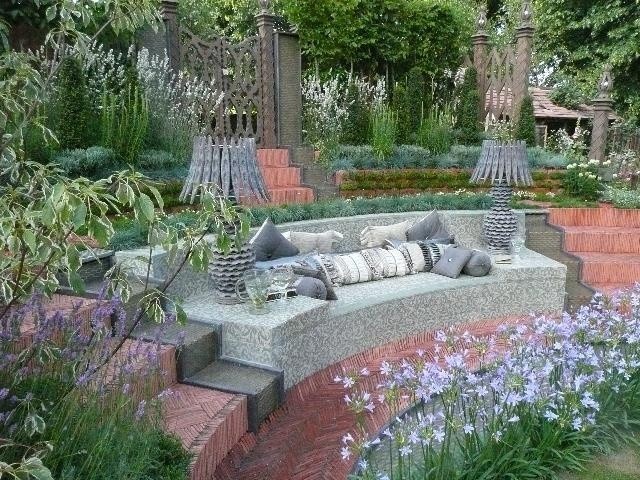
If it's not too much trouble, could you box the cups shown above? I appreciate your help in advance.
[235,268,271,316]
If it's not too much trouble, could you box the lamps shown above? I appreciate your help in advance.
[467,137,541,253]
[177,134,275,306]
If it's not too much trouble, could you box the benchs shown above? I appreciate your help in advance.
[104,204,571,394]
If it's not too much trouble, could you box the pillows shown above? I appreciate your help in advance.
[248,206,492,302]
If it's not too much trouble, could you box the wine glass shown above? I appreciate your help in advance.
[509,235,526,262]
[270,264,296,306]
